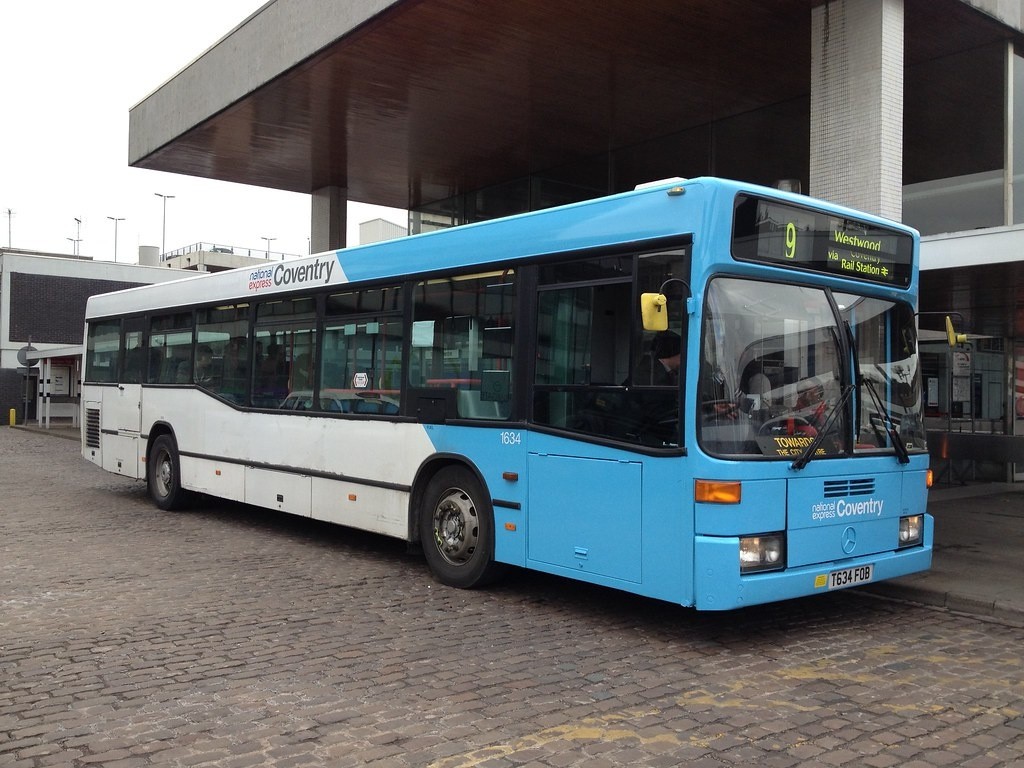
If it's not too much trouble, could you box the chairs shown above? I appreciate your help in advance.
[109,347,418,416]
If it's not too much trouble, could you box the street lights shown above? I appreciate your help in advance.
[74,217,81,256]
[155,193,175,261]
[108,217,126,263]
[261,237,277,259]
[67,237,83,255]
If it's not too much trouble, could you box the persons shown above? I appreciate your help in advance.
[126,330,316,408]
[721,329,772,412]
[614,330,739,430]
[789,376,839,436]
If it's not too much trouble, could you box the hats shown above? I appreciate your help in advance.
[796,376,824,398]
[652,329,681,359]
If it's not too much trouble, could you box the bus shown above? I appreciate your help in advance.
[80,176,967,613]
